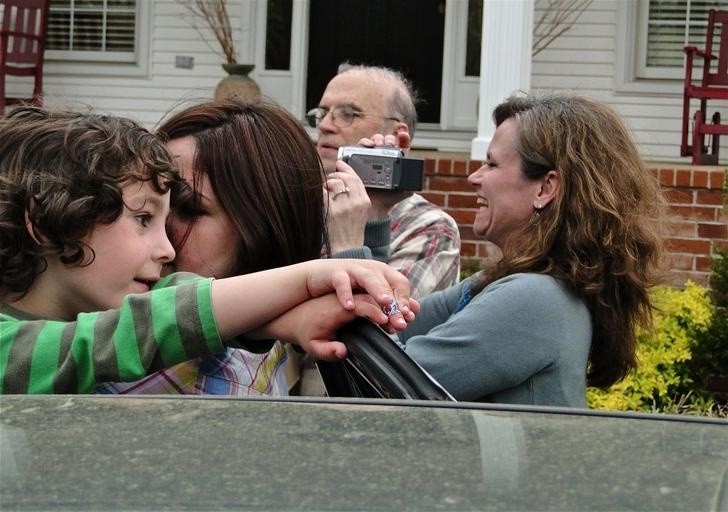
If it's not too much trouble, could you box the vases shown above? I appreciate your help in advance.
[216,63,261,105]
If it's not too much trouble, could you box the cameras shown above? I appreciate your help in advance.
[335,145,425,192]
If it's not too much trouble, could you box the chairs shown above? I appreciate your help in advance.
[680,8,728,157]
[1,1,50,119]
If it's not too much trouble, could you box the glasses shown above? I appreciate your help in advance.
[305,106,400,129]
